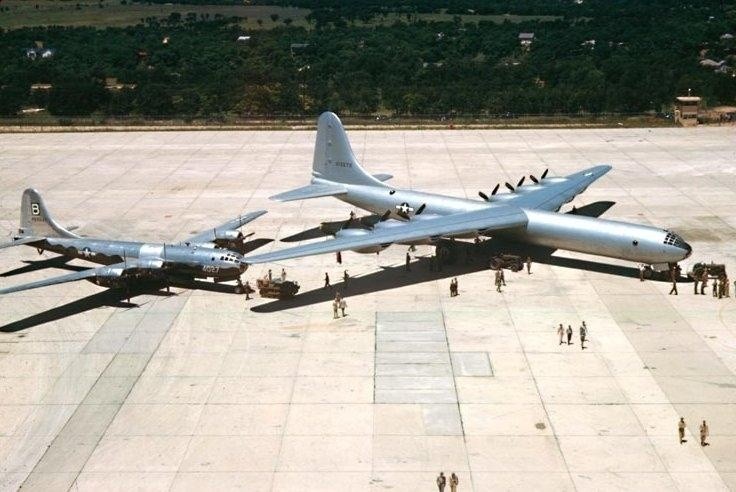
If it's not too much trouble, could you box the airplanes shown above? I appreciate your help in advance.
[234,106,695,280]
[0,186,271,305]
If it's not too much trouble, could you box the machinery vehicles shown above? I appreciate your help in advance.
[487,249,524,273]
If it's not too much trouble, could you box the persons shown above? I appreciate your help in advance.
[557,321,588,350]
[405,253,411,271]
[437,472,446,492]
[332,290,347,319]
[244,282,251,300]
[495,268,506,292]
[324,272,332,290]
[638,264,645,281]
[281,268,286,281]
[449,473,458,492]
[699,421,709,446]
[430,255,435,271]
[268,270,272,280]
[678,417,686,444]
[669,265,736,299]
[342,269,350,289]
[527,256,532,274]
[449,278,458,297]
